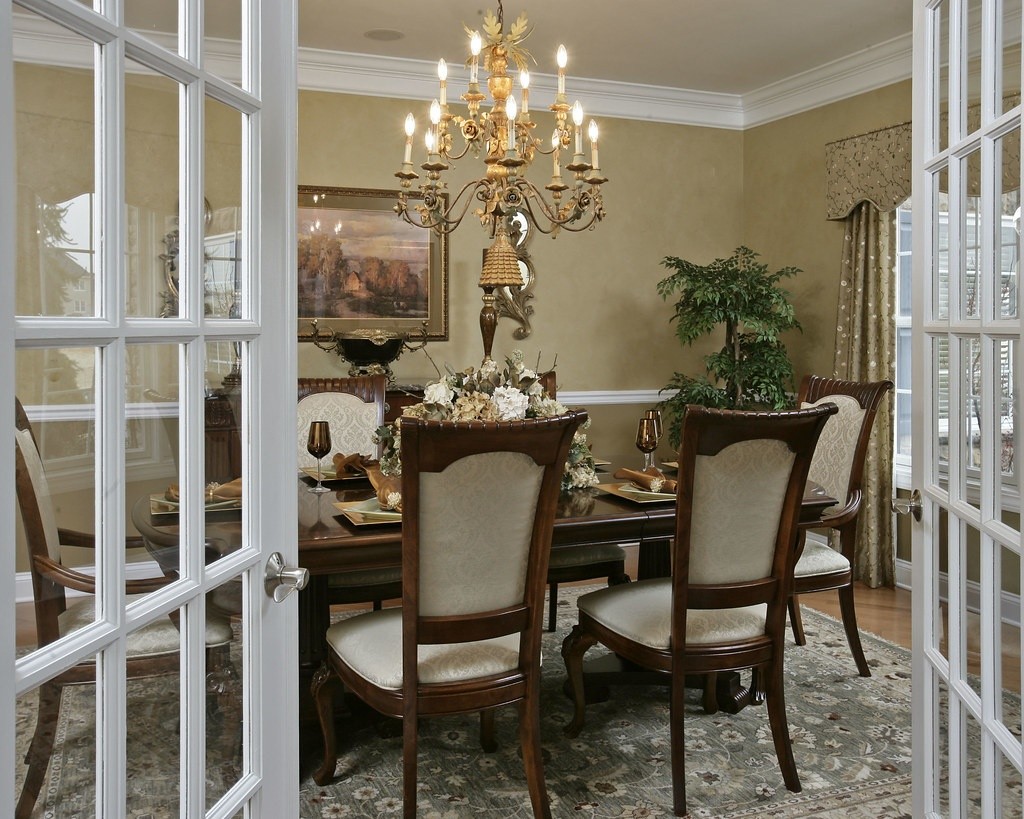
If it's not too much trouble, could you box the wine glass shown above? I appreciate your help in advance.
[635,418,659,471]
[306,420,332,493]
[644,409,664,468]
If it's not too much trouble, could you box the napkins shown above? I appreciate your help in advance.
[165,477,241,503]
[367,470,402,513]
[613,467,679,493]
[333,453,378,479]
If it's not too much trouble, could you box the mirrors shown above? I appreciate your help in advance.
[494,208,537,341]
[158,213,214,320]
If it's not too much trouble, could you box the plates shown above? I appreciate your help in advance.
[307,467,364,476]
[342,497,402,521]
[582,456,612,466]
[617,482,677,498]
[150,496,238,509]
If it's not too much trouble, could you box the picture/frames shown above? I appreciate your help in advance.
[297,184,449,342]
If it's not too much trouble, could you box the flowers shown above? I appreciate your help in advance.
[372,349,600,509]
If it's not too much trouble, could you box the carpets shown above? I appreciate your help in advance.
[14,605,1022,819]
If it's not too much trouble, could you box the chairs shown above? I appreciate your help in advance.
[789,374,895,678]
[16,398,243,818]
[522,372,632,632]
[311,408,588,819]
[561,402,839,816]
[298,375,402,651]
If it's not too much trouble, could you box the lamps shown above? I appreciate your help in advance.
[393,0,610,286]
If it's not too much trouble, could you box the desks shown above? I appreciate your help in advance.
[132,455,840,727]
[204,377,425,487]
